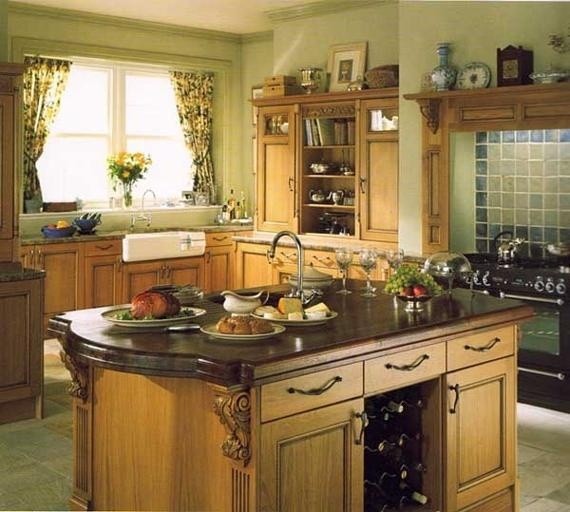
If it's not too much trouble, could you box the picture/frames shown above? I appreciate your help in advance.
[325,41,369,93]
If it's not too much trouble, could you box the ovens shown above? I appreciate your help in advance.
[491,288,570,380]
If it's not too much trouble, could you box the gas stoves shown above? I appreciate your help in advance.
[468,256,570,274]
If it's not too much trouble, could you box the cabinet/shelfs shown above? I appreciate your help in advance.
[1,264,48,427]
[0,61,24,264]
[247,339,366,512]
[366,321,520,511]
[17,224,253,343]
[232,235,395,295]
[246,87,399,243]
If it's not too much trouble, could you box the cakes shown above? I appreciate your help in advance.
[253,297,332,320]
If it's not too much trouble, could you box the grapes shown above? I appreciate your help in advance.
[386,263,442,294]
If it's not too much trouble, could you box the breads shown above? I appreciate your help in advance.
[130,292,180,318]
[216,316,272,335]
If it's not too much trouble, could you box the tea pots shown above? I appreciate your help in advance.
[307,189,332,204]
[491,231,524,262]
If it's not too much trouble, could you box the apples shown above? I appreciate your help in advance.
[413,284,426,296]
[400,287,413,296]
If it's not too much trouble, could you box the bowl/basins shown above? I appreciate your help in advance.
[41,226,77,237]
[73,219,99,229]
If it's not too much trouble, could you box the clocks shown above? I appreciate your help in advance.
[455,61,491,89]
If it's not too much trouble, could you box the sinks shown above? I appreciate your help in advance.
[207,289,287,309]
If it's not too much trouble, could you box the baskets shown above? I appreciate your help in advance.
[365,65,399,88]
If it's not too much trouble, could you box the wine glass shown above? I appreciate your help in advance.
[359,247,379,298]
[333,193,341,206]
[336,248,351,295]
[385,248,402,295]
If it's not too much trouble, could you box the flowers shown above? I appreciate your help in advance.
[105,149,151,207]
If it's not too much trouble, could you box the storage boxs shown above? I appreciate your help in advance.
[262,76,298,96]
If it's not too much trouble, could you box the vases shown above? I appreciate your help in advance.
[122,180,133,208]
[430,42,457,93]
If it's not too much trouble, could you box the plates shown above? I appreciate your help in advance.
[77,229,98,235]
[100,306,207,328]
[529,73,568,84]
[200,321,286,343]
[251,310,338,327]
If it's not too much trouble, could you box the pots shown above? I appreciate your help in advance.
[308,160,338,174]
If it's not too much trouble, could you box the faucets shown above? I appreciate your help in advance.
[138,189,156,227]
[265,229,306,305]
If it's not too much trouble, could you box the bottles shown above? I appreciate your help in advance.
[221,188,248,219]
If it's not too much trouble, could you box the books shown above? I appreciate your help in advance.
[304,118,356,146]
